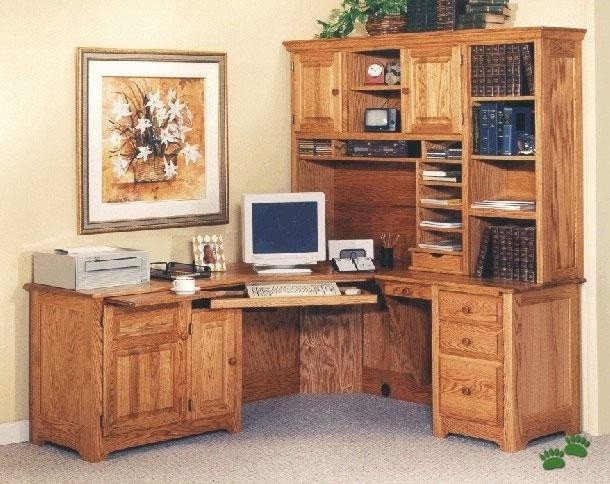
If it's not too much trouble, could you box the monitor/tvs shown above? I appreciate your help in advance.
[364,107,400,132]
[241,191,326,274]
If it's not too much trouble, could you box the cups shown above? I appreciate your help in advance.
[173,276,196,289]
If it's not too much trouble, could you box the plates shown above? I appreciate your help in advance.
[170,287,201,295]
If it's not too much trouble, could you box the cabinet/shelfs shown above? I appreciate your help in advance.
[24,27,589,464]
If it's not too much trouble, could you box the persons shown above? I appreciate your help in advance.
[203,244,216,263]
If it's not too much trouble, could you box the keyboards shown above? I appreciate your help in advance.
[245,283,340,299]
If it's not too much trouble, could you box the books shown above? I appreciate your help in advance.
[471,224,537,285]
[469,198,534,211]
[404,0,511,33]
[418,149,462,252]
[468,42,537,156]
[298,139,333,156]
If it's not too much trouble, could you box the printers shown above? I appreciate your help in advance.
[32,246,150,292]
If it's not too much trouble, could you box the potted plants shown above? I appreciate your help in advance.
[308,0,406,37]
